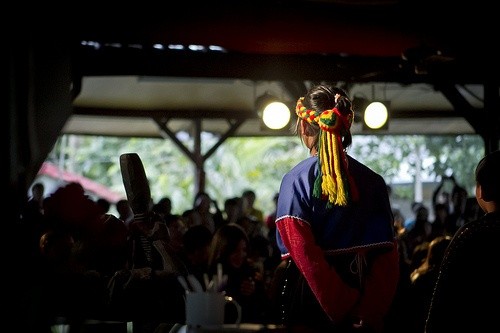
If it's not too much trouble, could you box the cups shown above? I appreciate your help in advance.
[184,290,242,333]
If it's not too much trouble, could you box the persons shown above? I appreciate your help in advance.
[275,84,399,333]
[18,150,500,333]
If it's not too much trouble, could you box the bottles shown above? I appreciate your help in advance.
[276,272,292,323]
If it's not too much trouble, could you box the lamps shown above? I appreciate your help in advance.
[352,97,389,131]
[256,92,292,131]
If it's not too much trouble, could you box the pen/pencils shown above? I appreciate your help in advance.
[177,263,228,293]
